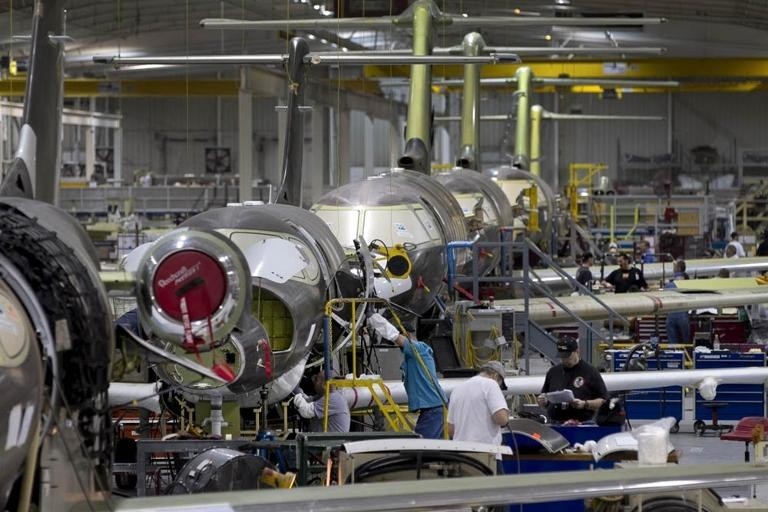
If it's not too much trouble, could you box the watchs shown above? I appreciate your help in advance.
[584,399,589,410]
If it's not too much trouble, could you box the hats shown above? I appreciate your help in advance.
[396,323,416,333]
[555,336,578,359]
[481,361,509,391]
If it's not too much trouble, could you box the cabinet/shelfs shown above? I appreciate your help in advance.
[713,319,747,343]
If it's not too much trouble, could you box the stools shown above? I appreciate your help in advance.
[720,416,768,462]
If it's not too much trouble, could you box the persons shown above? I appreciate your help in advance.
[537,336,608,425]
[575,252,594,295]
[717,228,768,321]
[673,261,689,279]
[639,241,654,264]
[665,271,691,344]
[366,310,448,439]
[284,370,352,480]
[447,360,510,478]
[600,252,650,294]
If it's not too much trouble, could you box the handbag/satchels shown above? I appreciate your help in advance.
[595,398,626,428]
